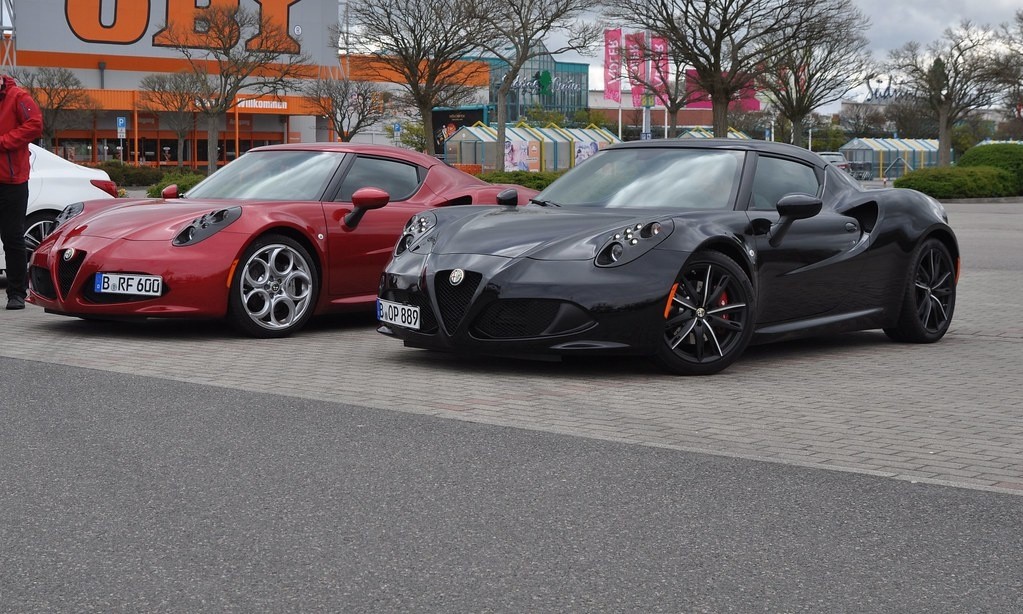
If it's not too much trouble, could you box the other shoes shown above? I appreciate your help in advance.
[6,294,26,309]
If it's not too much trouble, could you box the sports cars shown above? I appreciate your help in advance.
[376,136,962,377]
[22,142,547,340]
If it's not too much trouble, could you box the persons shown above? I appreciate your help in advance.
[0,74,43,309]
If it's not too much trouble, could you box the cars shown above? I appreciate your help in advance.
[816,151,851,174]
[0,143,118,280]
